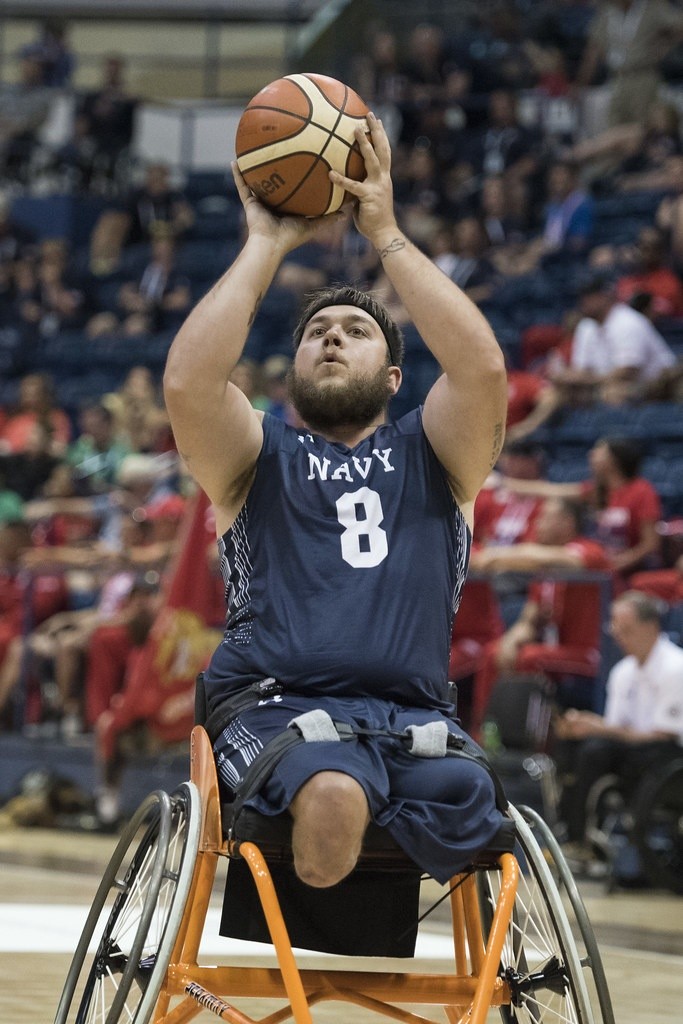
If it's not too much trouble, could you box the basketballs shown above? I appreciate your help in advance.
[229,68,376,227]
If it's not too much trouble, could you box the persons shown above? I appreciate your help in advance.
[1,0,683,872]
[164,111,506,889]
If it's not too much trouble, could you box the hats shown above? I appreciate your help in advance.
[141,495,185,523]
[565,266,608,298]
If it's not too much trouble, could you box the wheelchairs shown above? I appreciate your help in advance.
[570,755,683,901]
[50,674,615,1024]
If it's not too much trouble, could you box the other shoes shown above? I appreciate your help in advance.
[70,806,120,833]
[544,842,593,879]
